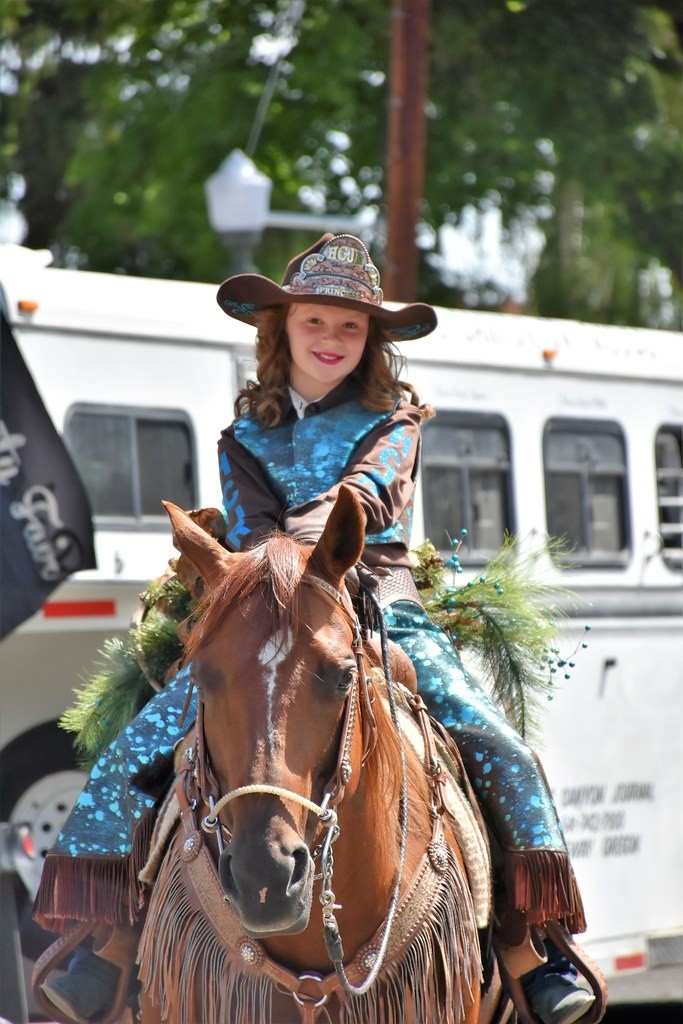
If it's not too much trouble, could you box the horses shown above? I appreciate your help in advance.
[131,484,515,1024]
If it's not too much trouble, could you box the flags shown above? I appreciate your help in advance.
[0,280,98,644]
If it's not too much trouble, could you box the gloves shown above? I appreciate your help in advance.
[297,540,380,598]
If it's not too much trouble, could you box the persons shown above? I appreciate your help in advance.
[31,234,598,1024]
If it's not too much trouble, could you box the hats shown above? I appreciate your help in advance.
[216,233,437,342]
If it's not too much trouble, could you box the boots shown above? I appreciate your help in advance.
[521,918,597,1024]
[40,936,121,1024]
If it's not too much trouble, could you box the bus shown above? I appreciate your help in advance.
[0,243,682,981]
